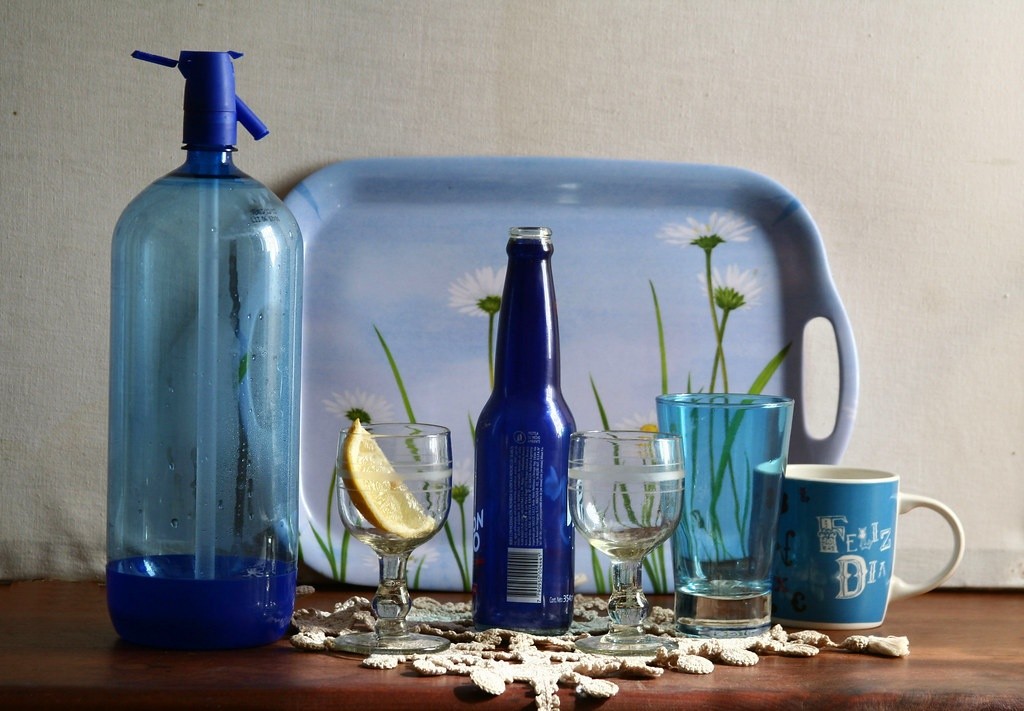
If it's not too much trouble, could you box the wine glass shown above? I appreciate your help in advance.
[336,423,453,654]
[567,430,685,656]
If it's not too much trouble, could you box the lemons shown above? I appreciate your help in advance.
[341,418,436,539]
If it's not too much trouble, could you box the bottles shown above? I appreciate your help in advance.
[106,51,302,649]
[474,226,577,638]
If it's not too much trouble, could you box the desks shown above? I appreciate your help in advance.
[0,576,1024,711]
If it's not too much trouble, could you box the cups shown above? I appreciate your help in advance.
[655,392,794,640]
[775,465,965,629]
